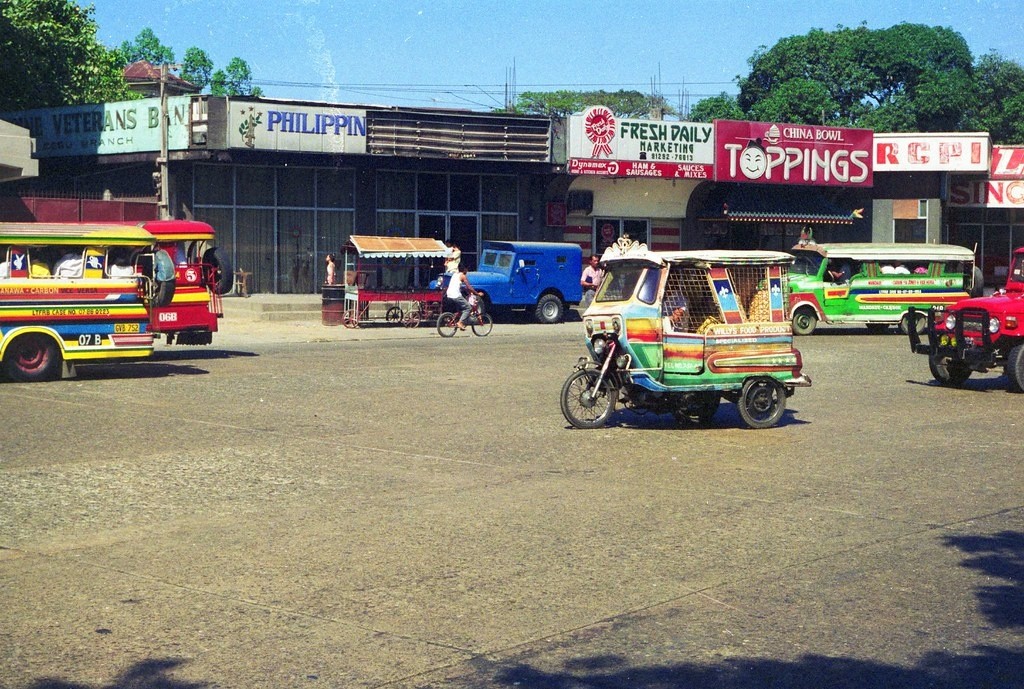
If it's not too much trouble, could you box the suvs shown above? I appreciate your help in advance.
[907,245,1024,396]
[429,241,582,324]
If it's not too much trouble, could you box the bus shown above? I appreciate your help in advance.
[0,221,233,384]
[779,225,983,337]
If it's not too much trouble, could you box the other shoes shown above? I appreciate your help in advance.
[456,321,466,331]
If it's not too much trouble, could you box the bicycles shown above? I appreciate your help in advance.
[437,287,492,337]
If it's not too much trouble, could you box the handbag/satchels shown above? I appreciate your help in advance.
[468,291,481,316]
[583,275,592,291]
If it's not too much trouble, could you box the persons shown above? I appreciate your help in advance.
[444,240,461,273]
[157,244,187,269]
[0,245,135,279]
[580,255,604,309]
[447,261,483,330]
[663,270,688,334]
[826,259,963,289]
[324,253,336,284]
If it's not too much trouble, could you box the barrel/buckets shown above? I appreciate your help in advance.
[322,284,348,326]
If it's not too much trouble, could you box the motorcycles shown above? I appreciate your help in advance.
[559,233,813,429]
